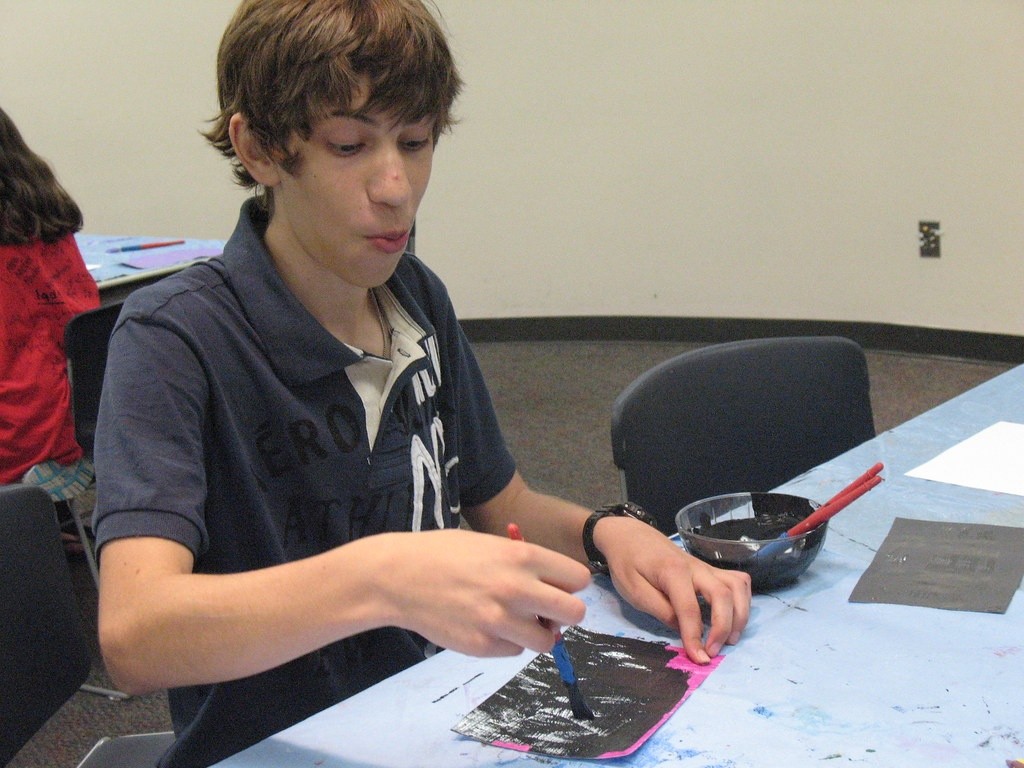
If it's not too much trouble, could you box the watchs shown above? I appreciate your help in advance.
[582,501,658,578]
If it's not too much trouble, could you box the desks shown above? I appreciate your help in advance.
[205,357,1024,768]
[74,232,228,296]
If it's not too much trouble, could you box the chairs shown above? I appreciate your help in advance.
[0,301,175,768]
[611,334,878,535]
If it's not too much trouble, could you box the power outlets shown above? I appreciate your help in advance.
[919,221,940,258]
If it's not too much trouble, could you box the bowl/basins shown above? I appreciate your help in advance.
[676,492,826,590]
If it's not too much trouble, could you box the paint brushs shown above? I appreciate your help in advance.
[746,462,884,563]
[105,239,185,254]
[508,524,593,720]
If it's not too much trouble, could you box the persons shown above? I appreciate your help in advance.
[94,0,752,696]
[0,109,101,502]
